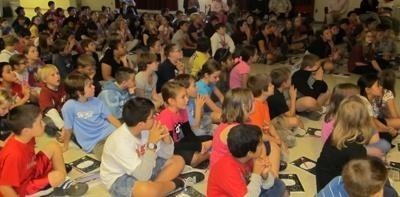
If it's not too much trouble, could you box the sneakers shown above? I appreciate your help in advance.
[279,160,287,171]
[53,178,89,196]
[173,169,205,192]
[297,111,322,120]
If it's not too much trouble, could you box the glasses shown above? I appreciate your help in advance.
[18,61,27,65]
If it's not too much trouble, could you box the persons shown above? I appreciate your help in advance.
[313,155,398,197]
[1,0,400,167]
[0,103,90,197]
[204,122,291,197]
[99,96,205,197]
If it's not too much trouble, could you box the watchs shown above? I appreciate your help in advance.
[146,142,158,150]
